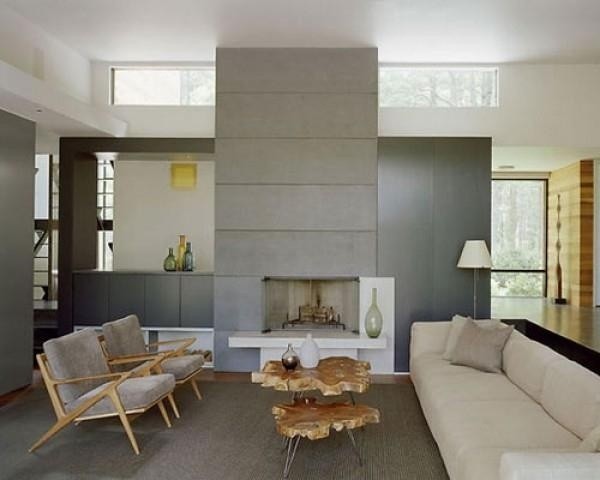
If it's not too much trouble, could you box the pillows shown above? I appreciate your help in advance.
[439,314,516,374]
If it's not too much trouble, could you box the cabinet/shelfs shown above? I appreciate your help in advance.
[72,268,214,328]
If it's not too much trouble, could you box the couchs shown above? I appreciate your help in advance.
[409,318,600,480]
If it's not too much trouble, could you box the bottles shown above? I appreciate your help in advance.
[176,235,186,271]
[164,247,177,272]
[298,333,321,369]
[182,242,193,272]
[281,343,299,371]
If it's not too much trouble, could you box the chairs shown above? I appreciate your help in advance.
[28,313,206,455]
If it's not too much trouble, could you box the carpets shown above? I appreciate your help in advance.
[0,376,448,480]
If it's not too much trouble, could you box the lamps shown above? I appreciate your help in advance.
[457,239,495,318]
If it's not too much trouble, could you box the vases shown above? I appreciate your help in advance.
[164,234,194,272]
[281,333,320,373]
